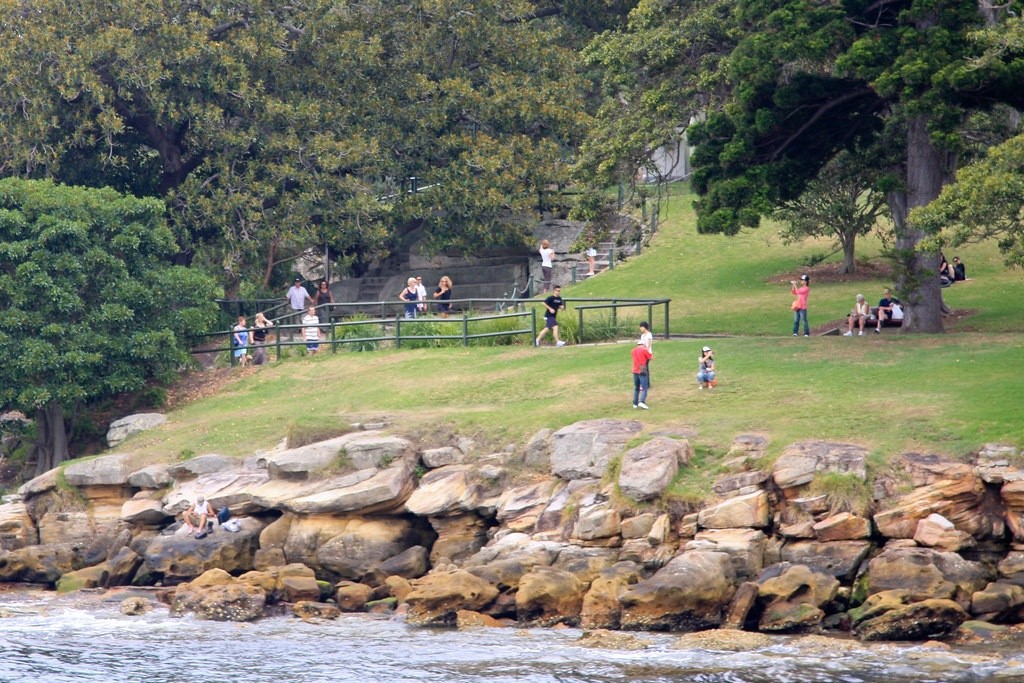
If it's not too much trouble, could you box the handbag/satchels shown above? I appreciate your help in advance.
[891,305,904,319]
[791,289,801,311]
[640,364,648,375]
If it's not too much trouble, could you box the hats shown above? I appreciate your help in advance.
[701,346,711,352]
[798,274,809,283]
[637,340,648,348]
[856,294,864,303]
[295,278,301,282]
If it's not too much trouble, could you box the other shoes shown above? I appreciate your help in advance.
[708,384,712,388]
[843,331,853,336]
[874,329,879,334]
[698,384,703,389]
[639,402,648,410]
[535,338,541,347]
[804,334,809,337]
[556,341,565,347]
[858,331,863,336]
[632,404,638,409]
[585,271,595,276]
[793,334,798,337]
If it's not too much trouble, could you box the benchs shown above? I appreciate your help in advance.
[847,307,904,327]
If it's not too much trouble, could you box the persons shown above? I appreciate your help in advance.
[696,346,716,389]
[410,275,428,317]
[313,280,336,333]
[538,240,556,294]
[399,277,421,321]
[286,279,313,333]
[949,257,965,282]
[433,276,453,318]
[301,307,320,357]
[535,285,567,349]
[233,316,249,366]
[630,340,652,410]
[940,253,949,281]
[636,322,654,390]
[843,294,872,336]
[585,235,596,276]
[248,313,273,364]
[790,275,810,337]
[875,288,903,334]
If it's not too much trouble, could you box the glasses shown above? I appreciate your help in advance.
[954,259,957,262]
[416,279,422,281]
[554,289,561,292]
